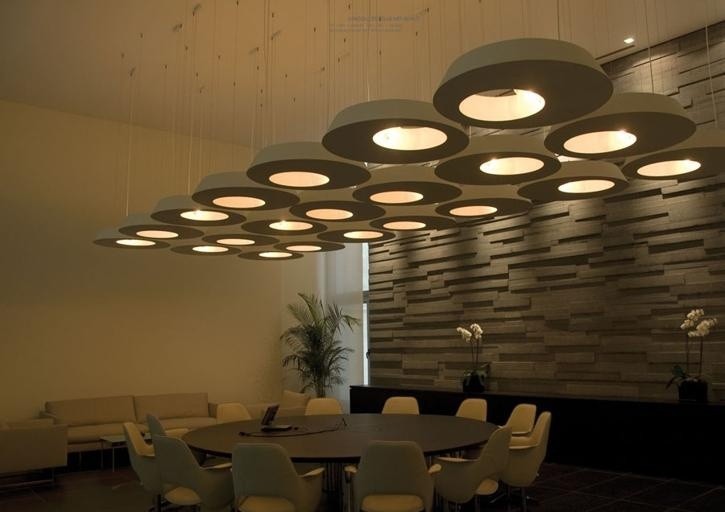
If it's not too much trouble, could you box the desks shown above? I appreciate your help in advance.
[183,413,499,463]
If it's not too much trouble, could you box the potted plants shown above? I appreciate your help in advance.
[455,321,487,394]
[273,291,367,398]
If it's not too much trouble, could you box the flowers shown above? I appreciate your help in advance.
[672,307,717,401]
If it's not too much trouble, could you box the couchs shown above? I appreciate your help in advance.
[0,416,68,489]
[235,387,310,419]
[41,392,248,471]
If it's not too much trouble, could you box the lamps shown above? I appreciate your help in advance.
[321,221,399,241]
[248,1,371,190]
[321,1,475,163]
[519,160,630,202]
[437,0,561,187]
[367,209,449,234]
[121,0,200,238]
[96,0,164,253]
[205,0,276,247]
[431,0,614,129]
[193,0,300,212]
[541,0,698,158]
[151,0,242,231]
[172,0,242,255]
[293,0,385,224]
[441,190,530,217]
[279,242,345,253]
[246,0,327,239]
[620,4,725,179]
[350,0,463,205]
[250,248,306,260]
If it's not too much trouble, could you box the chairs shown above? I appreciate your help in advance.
[122,421,200,512]
[146,413,205,459]
[455,398,488,423]
[305,398,343,415]
[153,434,237,512]
[217,403,251,422]
[381,396,421,416]
[342,438,441,512]
[485,403,535,438]
[474,411,553,512]
[231,442,325,512]
[436,424,513,512]
